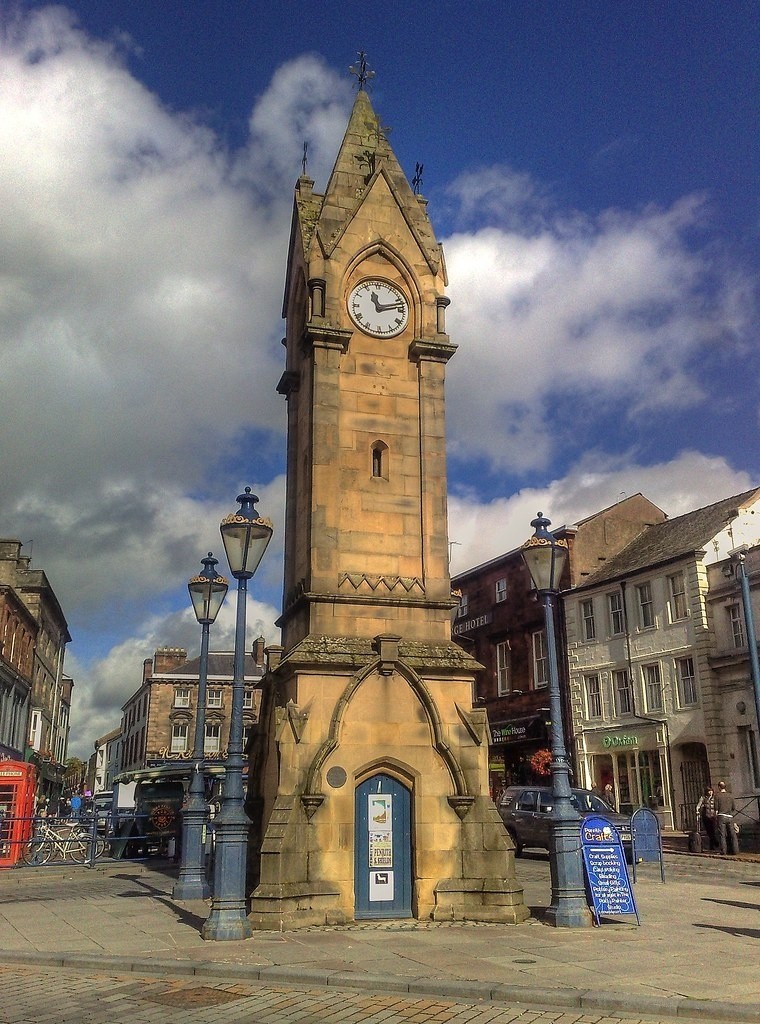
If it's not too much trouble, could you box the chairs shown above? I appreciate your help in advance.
[97,804,110,809]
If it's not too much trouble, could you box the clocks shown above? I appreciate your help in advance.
[347,281,409,339]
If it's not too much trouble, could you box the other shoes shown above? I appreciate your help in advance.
[714,840,719,848]
[709,846,715,850]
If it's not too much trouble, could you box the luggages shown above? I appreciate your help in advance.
[689,811,702,853]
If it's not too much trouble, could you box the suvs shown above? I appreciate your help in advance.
[497,785,640,865]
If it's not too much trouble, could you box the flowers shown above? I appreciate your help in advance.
[531,748,551,777]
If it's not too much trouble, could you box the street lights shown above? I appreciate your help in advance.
[727,544,759,732]
[518,512,593,929]
[171,551,228,901]
[200,487,274,942]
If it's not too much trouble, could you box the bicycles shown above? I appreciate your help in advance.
[22,819,99,865]
[31,820,105,860]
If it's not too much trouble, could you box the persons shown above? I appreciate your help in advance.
[696,781,740,855]
[37,792,86,823]
[590,782,614,806]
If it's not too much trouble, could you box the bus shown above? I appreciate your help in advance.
[91,791,113,803]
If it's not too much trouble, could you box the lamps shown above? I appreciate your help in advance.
[512,690,522,695]
[478,697,484,700]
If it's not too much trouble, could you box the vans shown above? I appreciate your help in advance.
[86,803,112,830]
